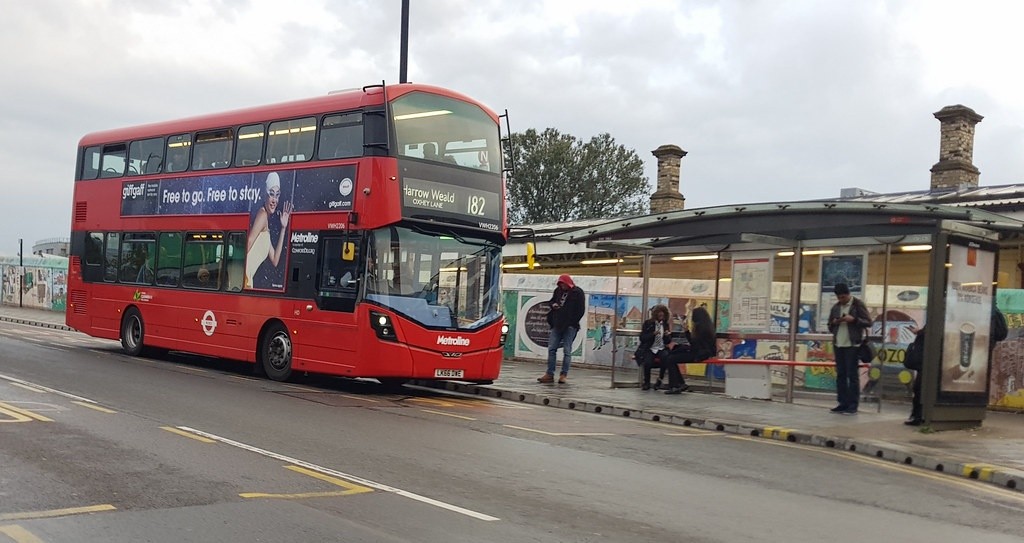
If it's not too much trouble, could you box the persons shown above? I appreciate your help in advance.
[636,303,718,395]
[423,144,437,158]
[905,322,927,424]
[826,284,874,416]
[537,274,586,385]
[91,234,217,290]
[245,172,292,292]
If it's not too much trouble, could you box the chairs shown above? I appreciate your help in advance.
[335,143,353,157]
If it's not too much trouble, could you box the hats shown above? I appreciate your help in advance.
[197,269,209,279]
[266,172,280,192]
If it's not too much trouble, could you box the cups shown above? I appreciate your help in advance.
[959,322,977,372]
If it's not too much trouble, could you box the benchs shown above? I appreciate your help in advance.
[630,354,872,404]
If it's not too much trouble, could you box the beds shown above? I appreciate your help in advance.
[106,158,258,179]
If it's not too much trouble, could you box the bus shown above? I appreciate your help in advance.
[66,83,538,388]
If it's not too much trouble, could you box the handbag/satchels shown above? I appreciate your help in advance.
[857,337,877,363]
[904,342,920,370]
[546,310,553,325]
[995,306,1008,341]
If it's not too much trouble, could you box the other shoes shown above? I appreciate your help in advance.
[847,406,857,413]
[904,414,921,426]
[679,383,686,390]
[664,387,682,394]
[654,378,662,390]
[642,381,651,390]
[831,405,846,412]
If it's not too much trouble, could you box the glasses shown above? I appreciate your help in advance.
[267,191,281,199]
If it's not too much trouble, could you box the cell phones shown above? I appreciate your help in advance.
[836,317,839,319]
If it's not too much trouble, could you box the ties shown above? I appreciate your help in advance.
[657,323,661,346]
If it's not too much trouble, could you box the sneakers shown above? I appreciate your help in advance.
[558,374,566,383]
[537,373,554,382]
